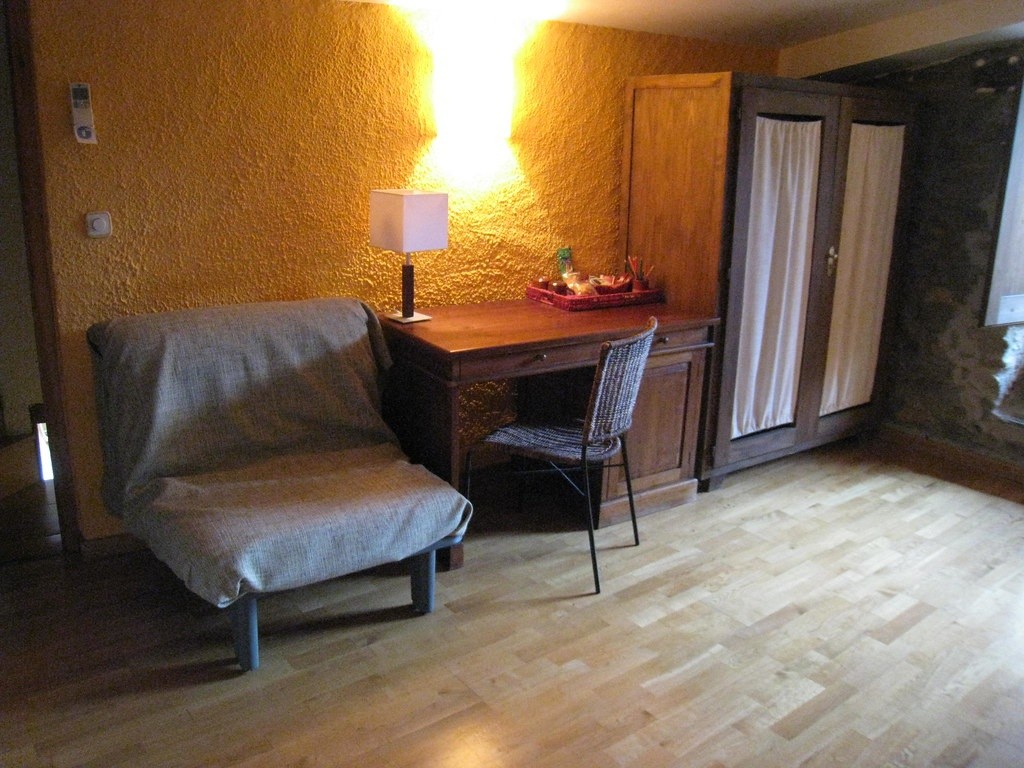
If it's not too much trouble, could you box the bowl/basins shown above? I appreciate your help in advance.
[594,272,632,294]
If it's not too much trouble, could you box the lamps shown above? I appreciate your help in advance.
[367,188,449,325]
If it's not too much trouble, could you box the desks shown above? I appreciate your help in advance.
[377,299,722,571]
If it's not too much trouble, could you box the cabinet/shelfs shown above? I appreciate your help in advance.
[618,70,927,492]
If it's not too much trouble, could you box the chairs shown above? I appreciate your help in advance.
[85,297,474,670]
[464,315,658,593]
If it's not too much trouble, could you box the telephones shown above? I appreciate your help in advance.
[71,83,98,144]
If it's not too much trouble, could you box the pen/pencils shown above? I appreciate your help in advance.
[624,256,654,280]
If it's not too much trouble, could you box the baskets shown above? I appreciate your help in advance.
[526,278,660,312]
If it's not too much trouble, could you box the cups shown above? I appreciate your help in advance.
[532,276,549,289]
[548,281,575,297]
[633,279,649,292]
